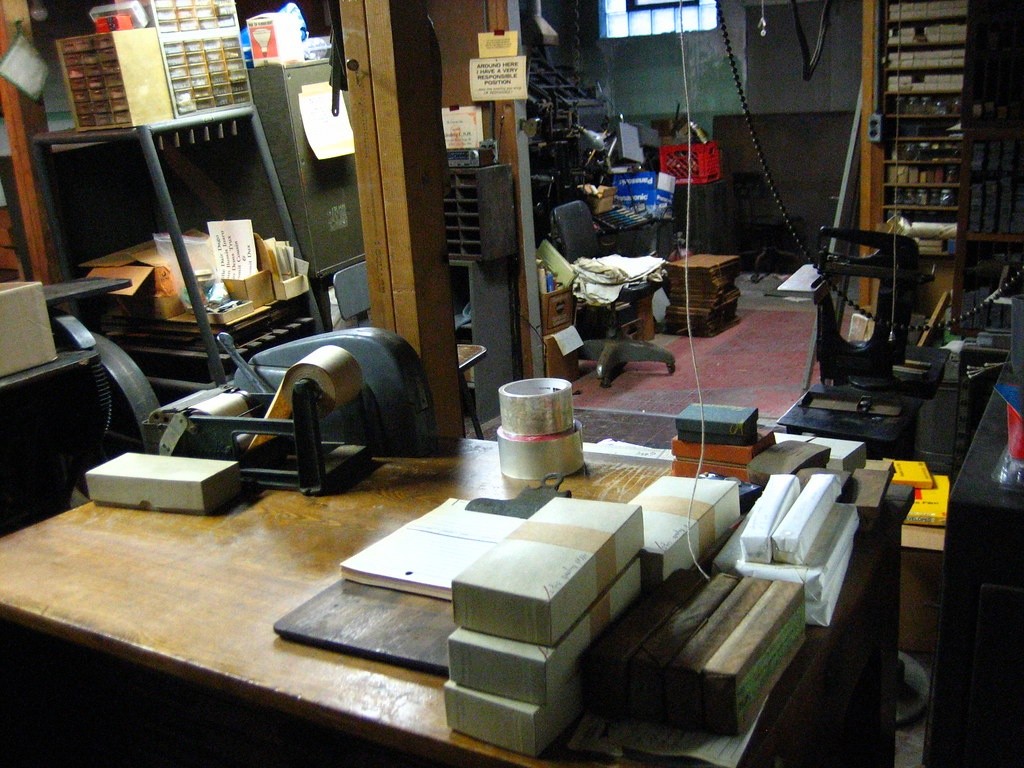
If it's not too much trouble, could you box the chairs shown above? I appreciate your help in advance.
[332,261,489,438]
[553,198,677,388]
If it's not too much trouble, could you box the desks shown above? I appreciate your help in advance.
[0,437,916,768]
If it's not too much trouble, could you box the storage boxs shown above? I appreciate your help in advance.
[901,526,945,653]
[577,183,617,214]
[78,228,209,319]
[453,496,642,647]
[805,437,867,476]
[670,429,775,469]
[447,555,643,707]
[269,272,307,300]
[221,269,275,308]
[676,402,761,446]
[245,13,304,66]
[538,283,573,335]
[444,669,593,758]
[0,281,58,378]
[542,333,580,383]
[672,460,748,485]
[630,475,741,585]
[659,140,721,186]
[83,451,239,514]
[613,172,676,220]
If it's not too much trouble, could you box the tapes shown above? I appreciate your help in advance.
[498,377,574,436]
[496,418,583,480]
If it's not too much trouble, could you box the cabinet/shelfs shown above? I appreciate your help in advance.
[443,162,518,262]
[858,0,1024,341]
[921,347,1023,768]
[0,0,372,392]
[448,259,513,424]
